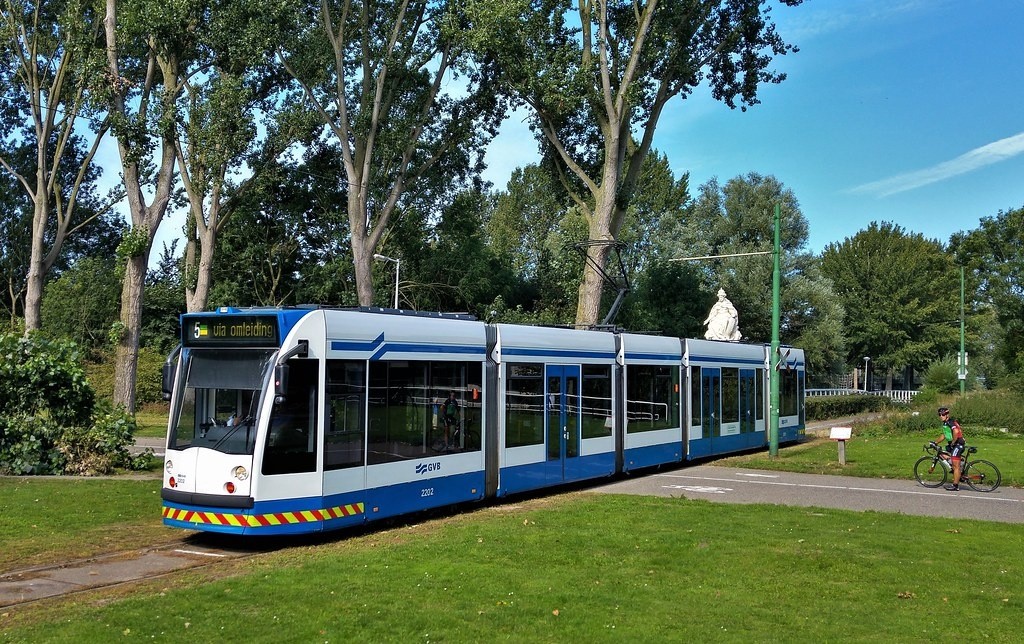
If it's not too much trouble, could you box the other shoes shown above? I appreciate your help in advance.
[949,467,954,473]
[945,483,959,491]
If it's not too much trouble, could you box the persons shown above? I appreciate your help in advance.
[517,404,536,444]
[227,392,251,427]
[440,390,462,451]
[707,285,737,341]
[932,407,966,491]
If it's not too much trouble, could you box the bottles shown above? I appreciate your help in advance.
[947,458,952,465]
[960,458,965,470]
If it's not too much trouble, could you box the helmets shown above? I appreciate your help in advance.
[938,408,949,414]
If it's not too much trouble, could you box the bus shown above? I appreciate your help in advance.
[160,242,806,536]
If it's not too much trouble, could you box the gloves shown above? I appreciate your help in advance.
[949,444,954,449]
[930,441,937,448]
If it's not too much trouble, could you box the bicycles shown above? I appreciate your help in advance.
[914,441,1001,492]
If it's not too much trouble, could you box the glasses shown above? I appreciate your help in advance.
[939,414,945,416]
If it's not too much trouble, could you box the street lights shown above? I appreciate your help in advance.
[863,357,870,394]
[372,253,400,309]
